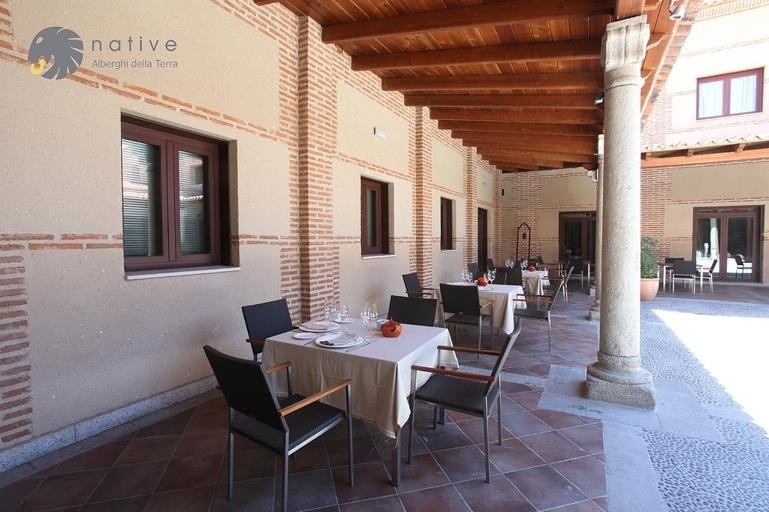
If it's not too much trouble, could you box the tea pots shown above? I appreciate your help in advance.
[381,317,402,338]
[529,265,535,271]
[477,277,488,286]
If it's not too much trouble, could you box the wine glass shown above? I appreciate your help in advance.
[484,270,496,290]
[462,272,473,285]
[361,303,379,341]
[504,258,528,273]
[324,302,349,329]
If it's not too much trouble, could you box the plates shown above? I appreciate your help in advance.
[315,334,363,347]
[299,321,340,332]
[295,332,314,338]
[334,318,352,323]
[479,288,491,291]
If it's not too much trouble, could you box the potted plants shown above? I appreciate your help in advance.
[641,234,660,301]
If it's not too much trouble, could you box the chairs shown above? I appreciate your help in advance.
[657,255,718,294]
[734,254,752,280]
